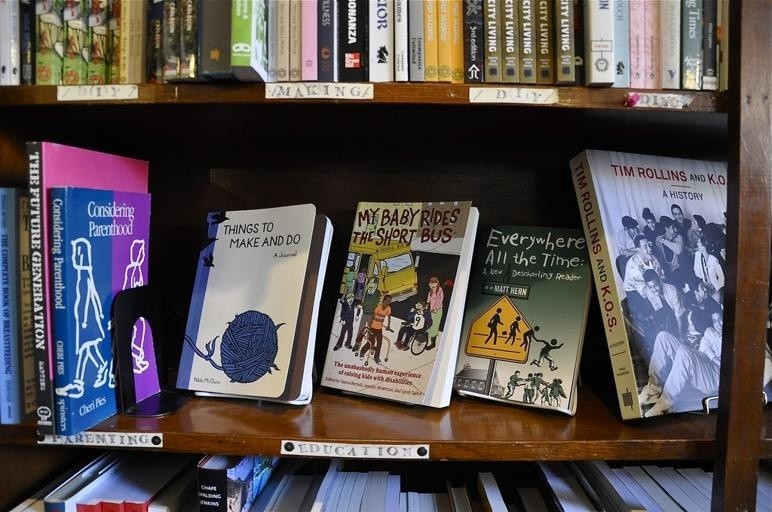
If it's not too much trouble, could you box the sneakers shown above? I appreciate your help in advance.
[638,383,663,405]
[644,399,670,417]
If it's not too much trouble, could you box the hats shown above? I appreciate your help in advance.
[621,216,639,230]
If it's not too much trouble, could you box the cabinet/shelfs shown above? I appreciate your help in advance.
[0,0,772,512]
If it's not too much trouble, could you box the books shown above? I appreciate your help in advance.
[8,452,772,511]
[2,133,731,440]
[1,0,731,92]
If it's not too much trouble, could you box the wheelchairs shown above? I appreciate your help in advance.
[396,308,434,356]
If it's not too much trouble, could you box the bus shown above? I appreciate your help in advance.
[341,237,419,306]
